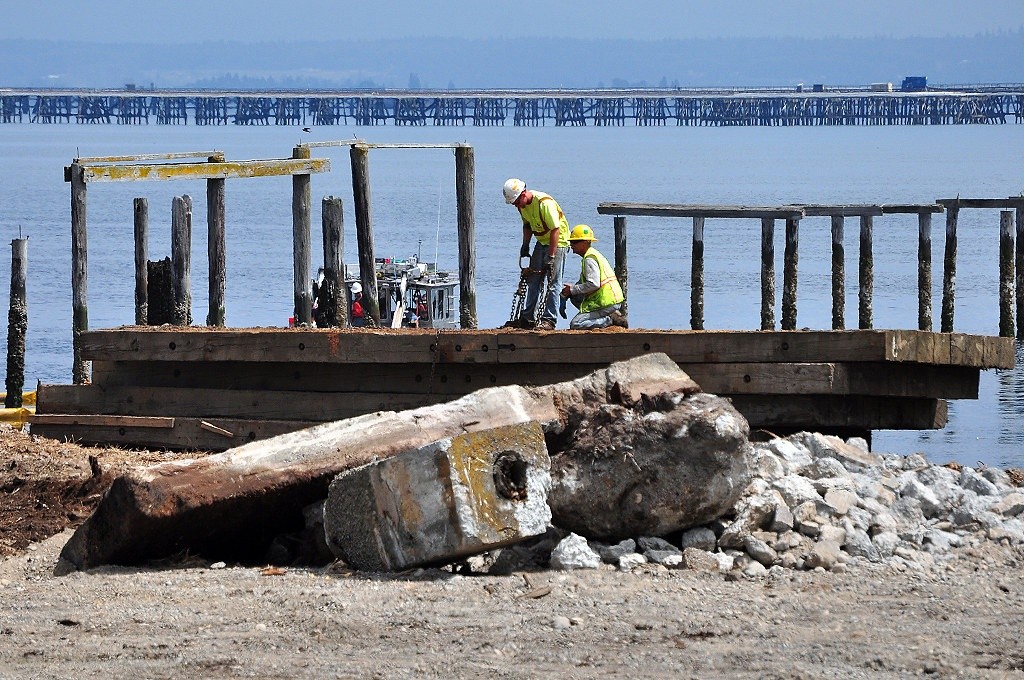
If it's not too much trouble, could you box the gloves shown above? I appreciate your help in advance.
[519,246,530,268]
[559,292,568,320]
[543,254,555,274]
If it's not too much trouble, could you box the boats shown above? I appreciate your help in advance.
[288,182,460,332]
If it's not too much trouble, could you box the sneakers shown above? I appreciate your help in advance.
[536,322,555,331]
[610,310,628,329]
[506,318,538,329]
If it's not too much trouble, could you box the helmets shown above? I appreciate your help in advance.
[350,282,362,293]
[502,178,525,204]
[567,224,598,242]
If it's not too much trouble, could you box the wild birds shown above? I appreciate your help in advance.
[302,127,313,133]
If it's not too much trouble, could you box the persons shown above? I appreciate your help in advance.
[561,224,629,330]
[502,178,571,331]
[349,281,366,326]
